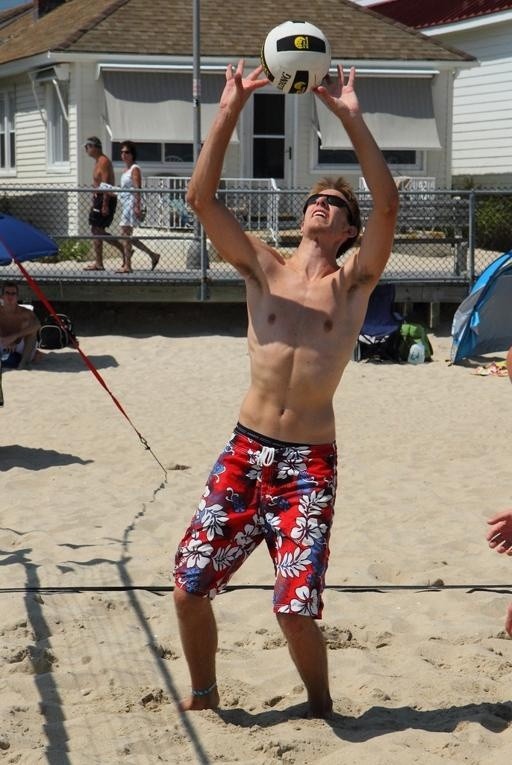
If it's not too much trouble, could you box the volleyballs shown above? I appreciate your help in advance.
[261,20,331,94]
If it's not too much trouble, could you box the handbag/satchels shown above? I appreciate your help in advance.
[37,310,73,349]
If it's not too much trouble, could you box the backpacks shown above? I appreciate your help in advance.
[402,324,433,362]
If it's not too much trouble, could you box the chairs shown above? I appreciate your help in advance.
[355,283,407,358]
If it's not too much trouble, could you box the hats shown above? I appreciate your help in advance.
[81,140,104,149]
[3,291,18,295]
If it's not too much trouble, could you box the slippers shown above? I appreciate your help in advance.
[83,249,160,274]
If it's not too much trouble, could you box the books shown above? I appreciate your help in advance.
[97,181,121,195]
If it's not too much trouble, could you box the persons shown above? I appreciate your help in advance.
[79,135,135,271]
[0,281,44,369]
[486,347,512,643]
[172,53,403,724]
[105,139,160,275]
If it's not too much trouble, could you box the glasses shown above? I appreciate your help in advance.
[119,148,133,155]
[302,193,358,228]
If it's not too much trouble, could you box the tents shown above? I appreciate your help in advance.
[449,247,512,366]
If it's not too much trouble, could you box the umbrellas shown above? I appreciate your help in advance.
[0,211,61,267]
